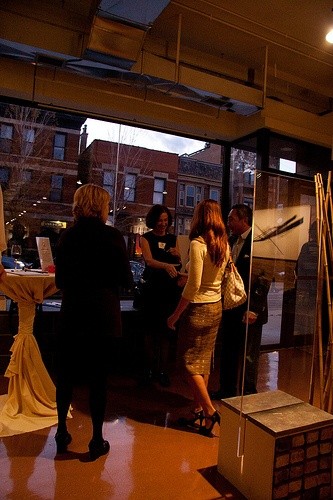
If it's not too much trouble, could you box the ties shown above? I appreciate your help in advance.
[232,237,243,265]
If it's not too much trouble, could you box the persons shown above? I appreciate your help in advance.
[210,204,271,400]
[140,204,182,392]
[55,184,134,460]
[168,199,231,433]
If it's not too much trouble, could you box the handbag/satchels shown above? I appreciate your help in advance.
[221,261,248,310]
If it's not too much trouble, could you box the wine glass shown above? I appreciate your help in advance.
[11,245,23,272]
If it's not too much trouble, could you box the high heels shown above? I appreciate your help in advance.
[89,440,110,461]
[55,431,72,451]
[199,410,221,434]
[178,409,205,427]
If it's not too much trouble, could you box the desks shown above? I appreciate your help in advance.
[0,268,63,437]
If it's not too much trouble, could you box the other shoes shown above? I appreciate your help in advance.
[159,365,170,386]
[210,389,236,399]
